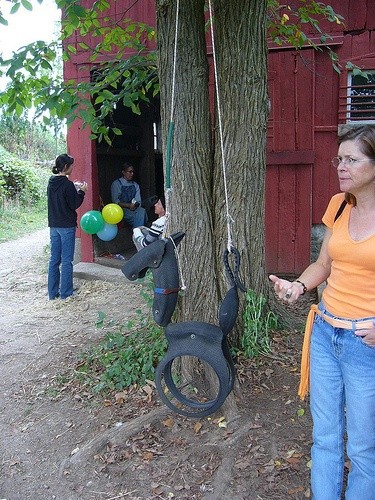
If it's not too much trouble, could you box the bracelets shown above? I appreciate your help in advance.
[296,280,306,293]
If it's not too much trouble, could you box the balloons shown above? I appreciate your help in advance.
[81,204,122,241]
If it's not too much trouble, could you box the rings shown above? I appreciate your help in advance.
[285,291,291,299]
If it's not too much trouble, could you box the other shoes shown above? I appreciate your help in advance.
[49,288,79,300]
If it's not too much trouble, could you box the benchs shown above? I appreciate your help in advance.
[93,221,135,256]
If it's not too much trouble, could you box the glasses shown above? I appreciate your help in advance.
[330,157,375,168]
[127,171,134,173]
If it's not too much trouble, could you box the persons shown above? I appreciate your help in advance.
[271,128,375,495]
[48,154,89,300]
[113,165,147,228]
[131,197,167,253]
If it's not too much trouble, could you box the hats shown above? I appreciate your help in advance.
[160,195,166,210]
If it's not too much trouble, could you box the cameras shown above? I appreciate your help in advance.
[74,182,83,190]
